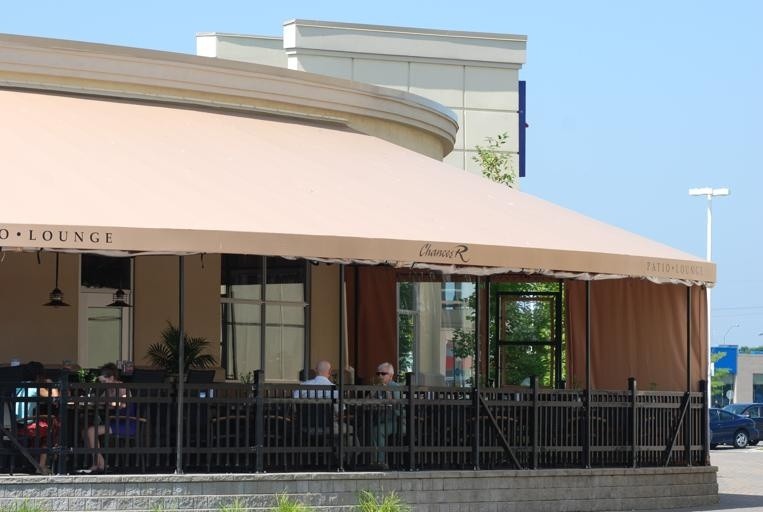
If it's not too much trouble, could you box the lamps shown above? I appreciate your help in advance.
[43,251,132,308]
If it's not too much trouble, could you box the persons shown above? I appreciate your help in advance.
[8,359,63,475]
[72,360,136,473]
[364,361,407,468]
[291,359,364,463]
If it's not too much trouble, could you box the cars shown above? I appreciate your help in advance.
[709,403,763,448]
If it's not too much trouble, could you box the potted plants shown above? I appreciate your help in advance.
[147,319,220,385]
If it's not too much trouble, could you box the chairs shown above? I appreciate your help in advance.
[1,383,681,475]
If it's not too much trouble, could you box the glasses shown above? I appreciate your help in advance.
[376,372,389,376]
